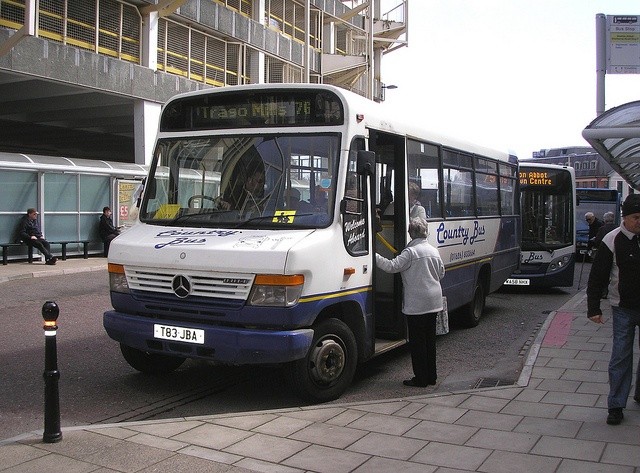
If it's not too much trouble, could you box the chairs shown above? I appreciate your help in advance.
[422,188,438,218]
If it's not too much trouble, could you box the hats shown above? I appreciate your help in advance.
[27,209,36,212]
[622,194,640,217]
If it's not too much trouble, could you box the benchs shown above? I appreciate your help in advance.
[0,240,107,266]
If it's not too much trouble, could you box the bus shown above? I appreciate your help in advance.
[575,187,620,259]
[503,161,577,287]
[102,80,523,401]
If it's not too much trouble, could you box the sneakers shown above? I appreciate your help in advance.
[411,376,436,385]
[607,409,625,425]
[49,258,58,262]
[46,261,56,265]
[403,379,428,387]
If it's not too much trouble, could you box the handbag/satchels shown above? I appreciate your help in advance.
[436,295,449,335]
[587,246,598,259]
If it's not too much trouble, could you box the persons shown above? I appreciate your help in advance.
[587,194,640,424]
[217,168,266,210]
[315,185,325,202]
[283,188,300,208]
[376,217,446,388]
[19,208,57,265]
[409,182,426,218]
[585,212,604,255]
[99,207,120,242]
[597,212,619,242]
